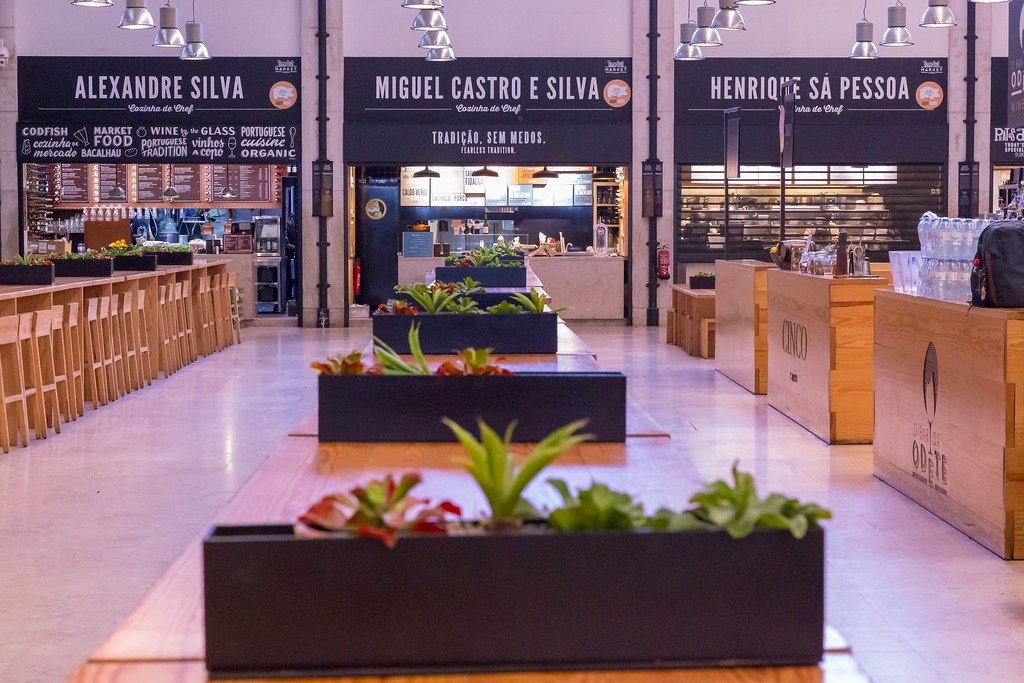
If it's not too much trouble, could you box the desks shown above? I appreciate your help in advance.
[871,288,1024,560]
[716,257,774,393]
[0,259,231,428]
[71,251,869,683]
[760,268,891,444]
[660,281,716,359]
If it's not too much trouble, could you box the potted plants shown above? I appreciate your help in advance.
[319,323,627,446]
[369,279,567,352]
[141,244,194,265]
[456,253,523,260]
[202,430,830,672]
[471,246,525,254]
[0,248,114,287]
[688,268,716,289]
[435,262,529,287]
[447,255,524,265]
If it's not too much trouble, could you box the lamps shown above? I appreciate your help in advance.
[108,164,123,197]
[472,166,498,177]
[163,164,180,196]
[413,166,439,178]
[219,164,238,198]
[674,1,775,61]
[401,0,456,61]
[532,165,559,180]
[844,0,877,59]
[880,0,914,46]
[70,0,211,60]
[919,0,957,29]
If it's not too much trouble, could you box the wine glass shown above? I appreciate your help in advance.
[461,223,466,234]
[467,219,473,234]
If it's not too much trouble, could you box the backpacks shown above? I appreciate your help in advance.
[970,221,1024,309]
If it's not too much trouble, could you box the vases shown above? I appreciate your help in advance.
[114,254,159,272]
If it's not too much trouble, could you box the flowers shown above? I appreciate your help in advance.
[108,239,132,255]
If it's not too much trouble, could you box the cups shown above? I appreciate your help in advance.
[82,207,156,221]
[443,243,450,257]
[909,251,922,294]
[206,240,212,253]
[80,214,84,233]
[497,239,503,246]
[434,243,441,257]
[888,250,909,293]
[56,213,80,233]
[178,235,189,243]
[214,240,220,254]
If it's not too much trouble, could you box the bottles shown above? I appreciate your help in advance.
[77,243,85,253]
[598,208,619,224]
[798,251,836,275]
[864,257,870,276]
[27,164,54,234]
[918,216,995,301]
[474,213,480,234]
[483,214,490,234]
[425,270,434,285]
[598,189,616,204]
[256,237,277,253]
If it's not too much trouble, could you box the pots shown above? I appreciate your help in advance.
[155,232,179,243]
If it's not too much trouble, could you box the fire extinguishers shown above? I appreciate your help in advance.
[352,257,361,295]
[658,243,670,279]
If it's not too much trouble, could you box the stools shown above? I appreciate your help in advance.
[0,271,243,455]
